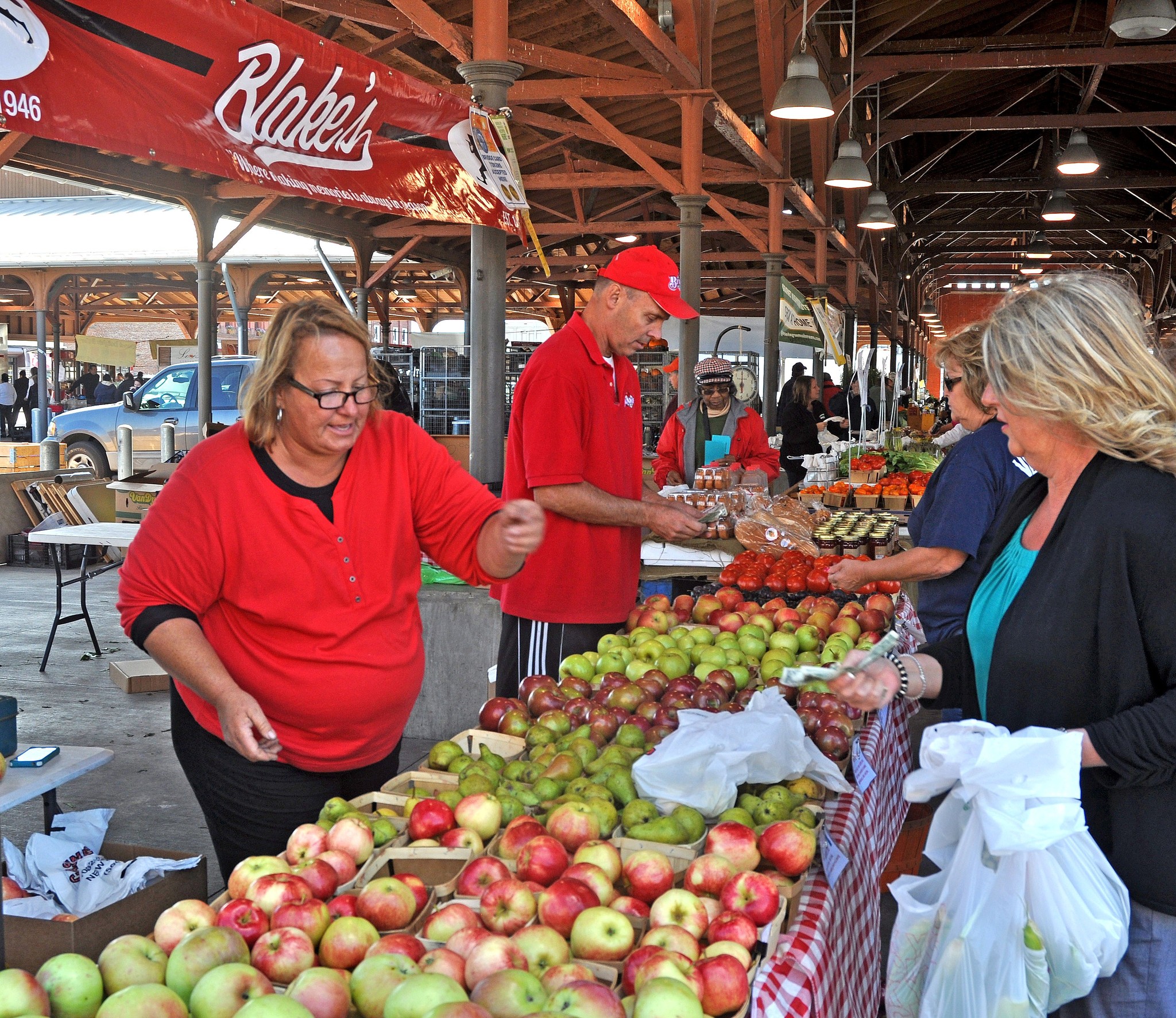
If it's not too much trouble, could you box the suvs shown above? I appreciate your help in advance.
[47,353,261,483]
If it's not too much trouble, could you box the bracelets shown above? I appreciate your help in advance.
[899,654,926,701]
[883,653,908,700]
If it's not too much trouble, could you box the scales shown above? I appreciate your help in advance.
[711,325,759,406]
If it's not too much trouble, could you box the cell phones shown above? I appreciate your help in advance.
[10,746,60,767]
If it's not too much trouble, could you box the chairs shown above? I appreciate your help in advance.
[211,376,238,406]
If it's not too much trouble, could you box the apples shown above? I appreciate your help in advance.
[559,627,877,694]
[627,595,895,644]
[715,587,744,610]
[478,676,862,763]
[0,789,815,1018]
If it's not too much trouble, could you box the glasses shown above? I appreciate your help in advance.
[886,384,894,390]
[700,385,729,395]
[944,374,965,391]
[284,374,380,410]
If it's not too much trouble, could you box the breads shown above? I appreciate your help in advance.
[734,496,831,559]
[663,468,766,539]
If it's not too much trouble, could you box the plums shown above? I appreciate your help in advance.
[692,582,893,610]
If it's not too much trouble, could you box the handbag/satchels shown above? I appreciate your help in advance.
[922,780,1131,1012]
[884,789,1049,1018]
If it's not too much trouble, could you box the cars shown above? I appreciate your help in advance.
[378,347,394,353]
[396,347,457,355]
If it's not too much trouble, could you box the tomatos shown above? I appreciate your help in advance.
[800,454,933,507]
[718,550,901,594]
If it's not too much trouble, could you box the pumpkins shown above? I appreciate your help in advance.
[639,338,668,388]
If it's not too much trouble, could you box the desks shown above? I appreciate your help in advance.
[28,522,139,672]
[212,558,927,1018]
[0,743,115,836]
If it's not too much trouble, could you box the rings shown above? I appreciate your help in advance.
[725,463,728,466]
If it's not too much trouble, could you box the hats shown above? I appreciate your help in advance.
[792,362,807,371]
[662,357,679,373]
[694,358,733,385]
[595,244,701,320]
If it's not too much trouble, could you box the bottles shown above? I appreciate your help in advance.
[77,394,86,400]
[71,395,75,399]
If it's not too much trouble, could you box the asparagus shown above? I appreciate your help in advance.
[904,441,943,461]
[885,430,902,451]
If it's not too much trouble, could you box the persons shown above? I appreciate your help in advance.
[896,401,908,427]
[828,323,1039,721]
[823,373,843,416]
[650,357,781,492]
[928,397,974,449]
[828,271,1176,1018]
[0,367,55,438]
[488,247,706,696]
[659,357,679,435]
[829,372,881,441]
[66,364,100,405]
[869,377,894,421]
[777,362,807,427]
[93,372,148,405]
[118,300,541,888]
[780,376,849,488]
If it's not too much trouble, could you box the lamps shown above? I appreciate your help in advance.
[1057,126,1101,175]
[1019,259,1044,274]
[768,0,837,119]
[855,80,896,230]
[1109,0,1176,39]
[1025,231,1053,259]
[811,0,873,189]
[1041,188,1076,222]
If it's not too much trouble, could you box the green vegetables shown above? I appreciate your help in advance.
[839,446,882,476]
[882,450,941,473]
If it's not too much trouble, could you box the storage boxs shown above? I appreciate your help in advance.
[106,462,180,566]
[346,726,710,996]
[3,841,208,974]
[907,403,934,430]
[5,532,97,570]
[109,658,170,693]
[639,541,734,567]
[798,469,923,511]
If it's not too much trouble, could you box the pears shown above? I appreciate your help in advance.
[316,725,819,845]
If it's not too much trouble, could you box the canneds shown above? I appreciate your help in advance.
[812,511,899,559]
